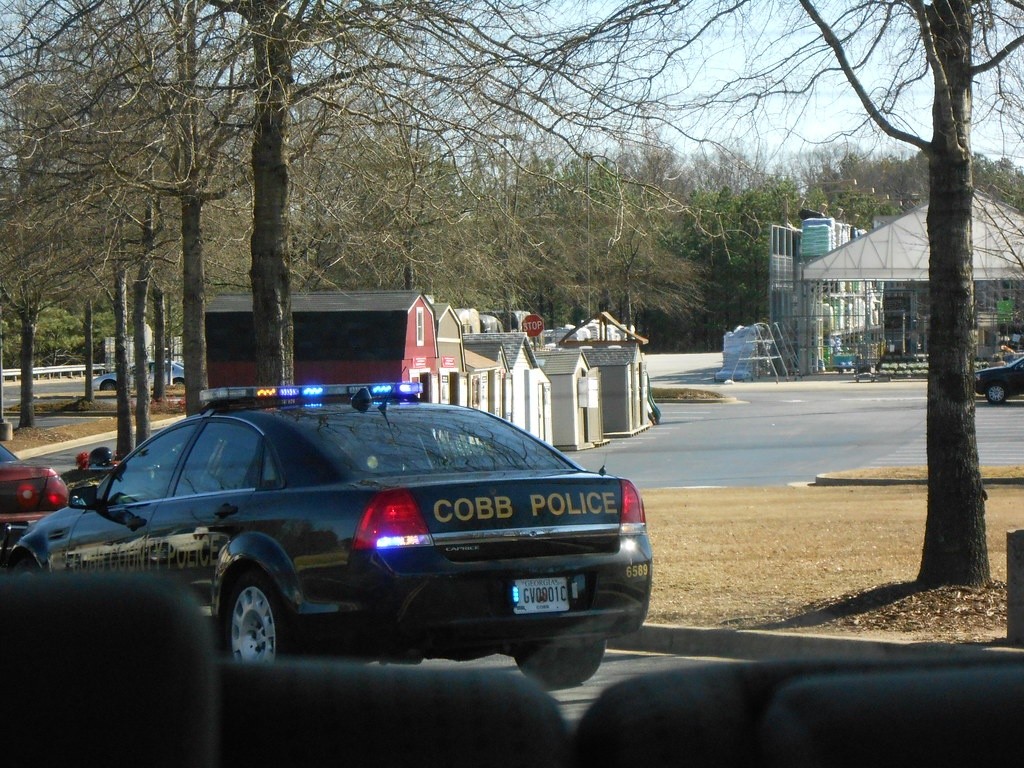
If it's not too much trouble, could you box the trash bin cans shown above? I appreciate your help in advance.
[1006,529,1024,648]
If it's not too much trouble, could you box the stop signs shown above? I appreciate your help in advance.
[522,313,543,337]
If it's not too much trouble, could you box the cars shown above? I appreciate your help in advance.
[7,385,655,688]
[0,439,68,561]
[970,356,1024,405]
[92,361,188,393]
